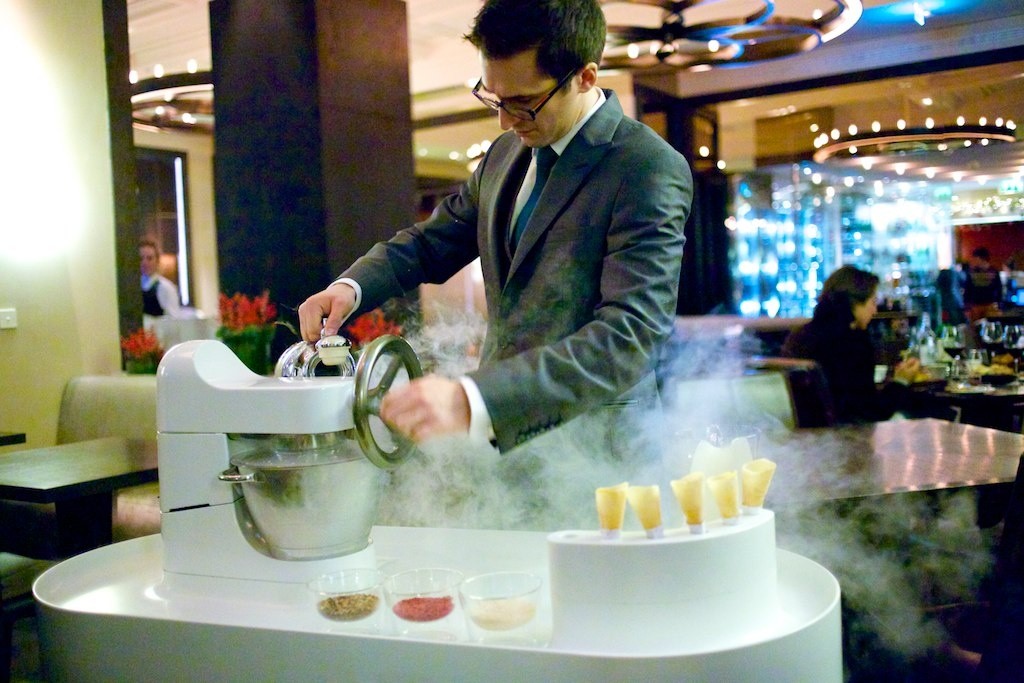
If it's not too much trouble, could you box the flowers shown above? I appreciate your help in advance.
[349,310,400,345]
[217,289,274,372]
[119,327,164,373]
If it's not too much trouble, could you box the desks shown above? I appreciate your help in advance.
[0,436,159,561]
[796,418,1024,683]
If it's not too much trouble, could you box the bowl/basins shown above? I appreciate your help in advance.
[458,571,544,630]
[383,567,458,621]
[218,441,379,561]
[308,569,382,621]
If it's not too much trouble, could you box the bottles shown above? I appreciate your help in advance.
[919,312,935,369]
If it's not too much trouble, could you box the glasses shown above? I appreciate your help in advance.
[472,66,580,122]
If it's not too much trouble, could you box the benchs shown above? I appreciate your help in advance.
[34,376,160,540]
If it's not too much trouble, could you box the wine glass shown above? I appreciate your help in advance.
[1003,325,1024,373]
[942,326,966,380]
[980,321,1005,358]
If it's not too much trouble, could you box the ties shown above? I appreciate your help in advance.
[510,144,560,258]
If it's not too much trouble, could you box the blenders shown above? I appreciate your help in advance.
[155,318,424,599]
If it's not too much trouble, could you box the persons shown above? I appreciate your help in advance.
[962,246,1002,318]
[135,237,180,317]
[297,0,693,456]
[807,266,929,426]
